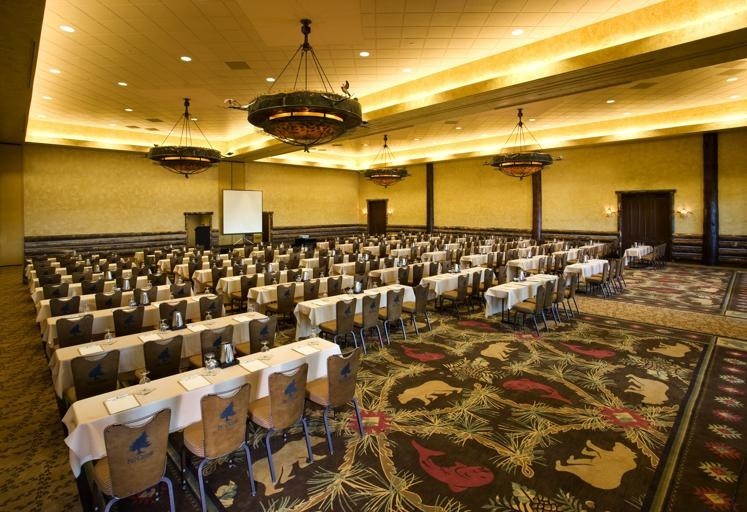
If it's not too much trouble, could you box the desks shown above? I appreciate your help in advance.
[59,337,343,476]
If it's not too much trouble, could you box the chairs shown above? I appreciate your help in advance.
[93,407,175,511]
[304,346,362,455]
[180,384,258,511]
[22,228,666,404]
[249,364,311,481]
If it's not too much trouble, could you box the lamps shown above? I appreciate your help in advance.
[224,18,362,153]
[491,108,553,182]
[363,134,410,188]
[136,97,222,179]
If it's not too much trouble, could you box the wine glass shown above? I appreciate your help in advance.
[205,352,217,376]
[138,370,153,393]
[161,319,169,333]
[204,286,210,296]
[104,328,113,343]
[272,277,278,288]
[320,271,324,279]
[260,341,271,359]
[206,311,213,322]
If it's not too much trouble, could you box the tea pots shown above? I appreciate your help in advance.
[75,254,113,282]
[518,270,526,281]
[171,311,184,329]
[303,272,309,283]
[139,290,150,305]
[354,281,364,295]
[123,278,130,291]
[220,341,234,364]
[453,263,460,272]
